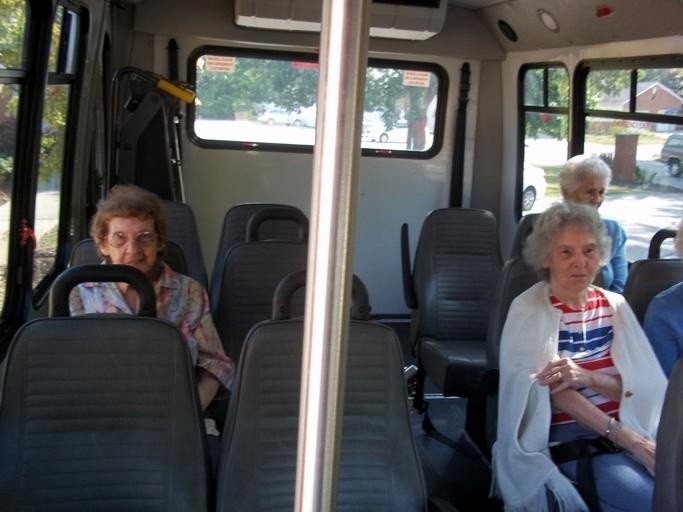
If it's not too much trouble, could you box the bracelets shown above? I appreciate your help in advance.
[604,418,614,439]
[613,423,623,447]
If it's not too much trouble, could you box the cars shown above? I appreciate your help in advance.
[361,120,429,143]
[255,110,300,127]
[521,162,548,211]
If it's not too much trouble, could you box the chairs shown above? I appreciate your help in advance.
[0,204,683,511]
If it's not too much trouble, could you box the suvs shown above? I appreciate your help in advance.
[660,134,682,176]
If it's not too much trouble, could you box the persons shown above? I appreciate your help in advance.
[490,199,669,512]
[66,185,237,476]
[558,154,629,293]
[643,216,683,379]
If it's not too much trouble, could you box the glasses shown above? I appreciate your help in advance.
[102,231,157,248]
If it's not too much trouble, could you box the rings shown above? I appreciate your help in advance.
[558,371,562,381]
